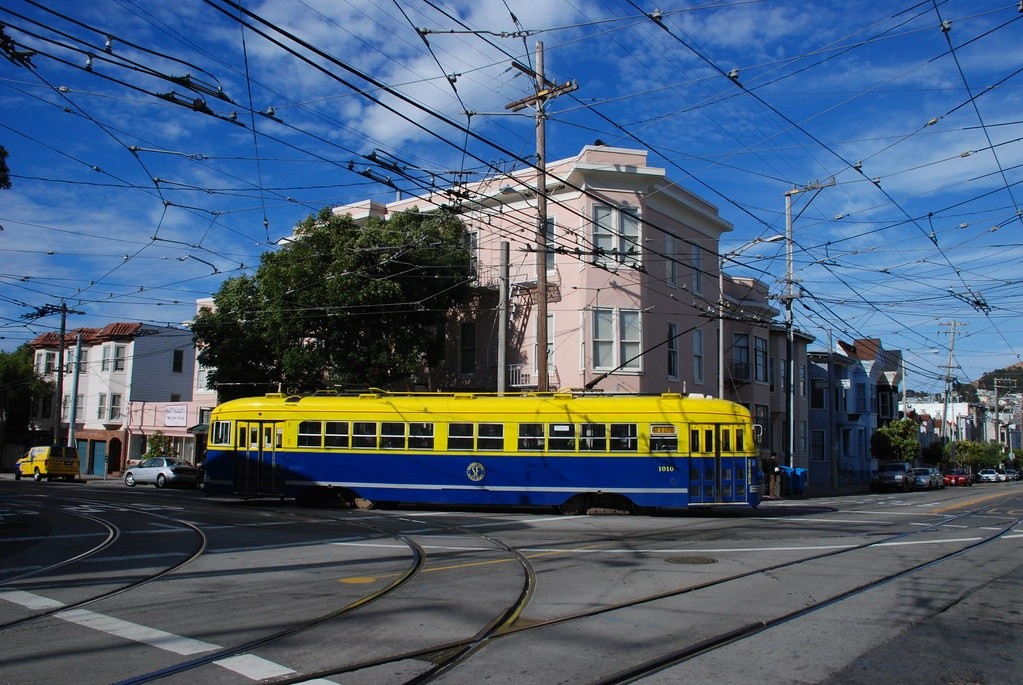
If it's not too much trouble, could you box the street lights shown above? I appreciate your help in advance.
[720,235,786,399]
[500,184,551,394]
[901,349,940,421]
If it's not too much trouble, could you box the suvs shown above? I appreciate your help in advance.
[872,462,915,491]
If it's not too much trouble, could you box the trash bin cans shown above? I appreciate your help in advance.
[780,465,808,497]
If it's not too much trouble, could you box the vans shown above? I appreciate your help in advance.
[14,446,80,481]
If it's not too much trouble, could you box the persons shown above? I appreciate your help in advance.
[765,452,781,498]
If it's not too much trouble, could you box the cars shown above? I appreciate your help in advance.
[122,456,201,488]
[1005,469,1019,480]
[979,469,999,482]
[945,470,972,486]
[912,467,943,490]
[996,470,1008,482]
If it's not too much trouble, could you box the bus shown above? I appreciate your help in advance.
[202,388,763,517]
